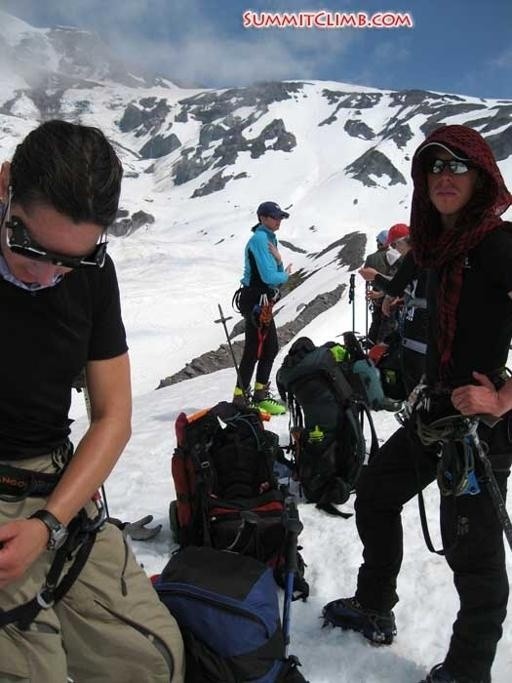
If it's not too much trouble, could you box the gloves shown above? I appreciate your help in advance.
[105,515,164,542]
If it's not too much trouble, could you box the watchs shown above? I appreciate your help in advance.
[30,510,69,552]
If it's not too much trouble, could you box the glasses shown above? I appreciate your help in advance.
[4,183,108,272]
[423,156,473,176]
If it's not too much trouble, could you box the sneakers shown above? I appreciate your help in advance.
[321,595,396,645]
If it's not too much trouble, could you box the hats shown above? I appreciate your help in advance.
[376,224,411,245]
[257,201,289,219]
[416,139,466,159]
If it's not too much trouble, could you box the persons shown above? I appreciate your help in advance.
[234,201,292,421]
[1,119,188,682]
[364,230,389,348]
[322,124,511,683]
[358,223,414,318]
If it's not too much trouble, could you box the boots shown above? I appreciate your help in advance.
[253,380,288,416]
[232,386,270,422]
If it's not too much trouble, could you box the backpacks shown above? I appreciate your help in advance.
[149,544,309,683]
[370,306,432,412]
[276,337,378,519]
[169,401,310,602]
